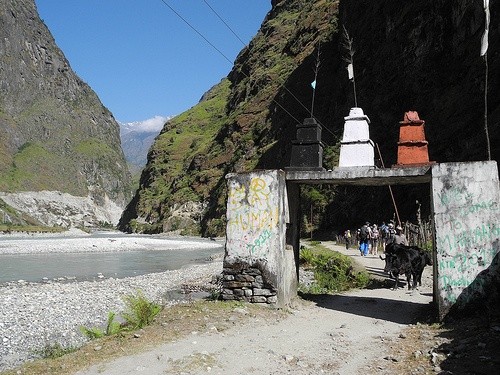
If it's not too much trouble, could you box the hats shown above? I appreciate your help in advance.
[397,227,402,231]
[388,224,394,228]
[365,222,370,224]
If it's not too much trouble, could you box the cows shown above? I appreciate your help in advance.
[379,242,433,294]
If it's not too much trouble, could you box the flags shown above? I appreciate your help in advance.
[480,0,491,56]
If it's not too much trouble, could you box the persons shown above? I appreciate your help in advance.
[344,220,408,259]
